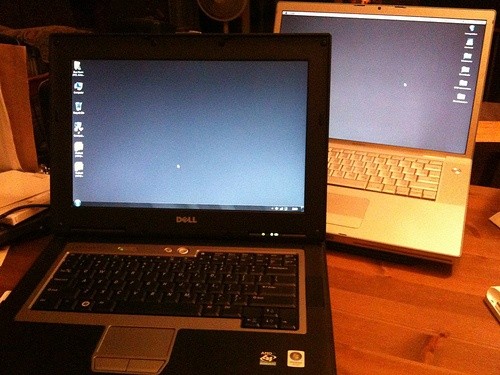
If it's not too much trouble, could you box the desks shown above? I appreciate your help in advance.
[1,184,500,374]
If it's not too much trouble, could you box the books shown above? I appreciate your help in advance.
[0,168,52,226]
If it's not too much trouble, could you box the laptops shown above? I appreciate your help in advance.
[0,33,336,375]
[272,2,496,265]
